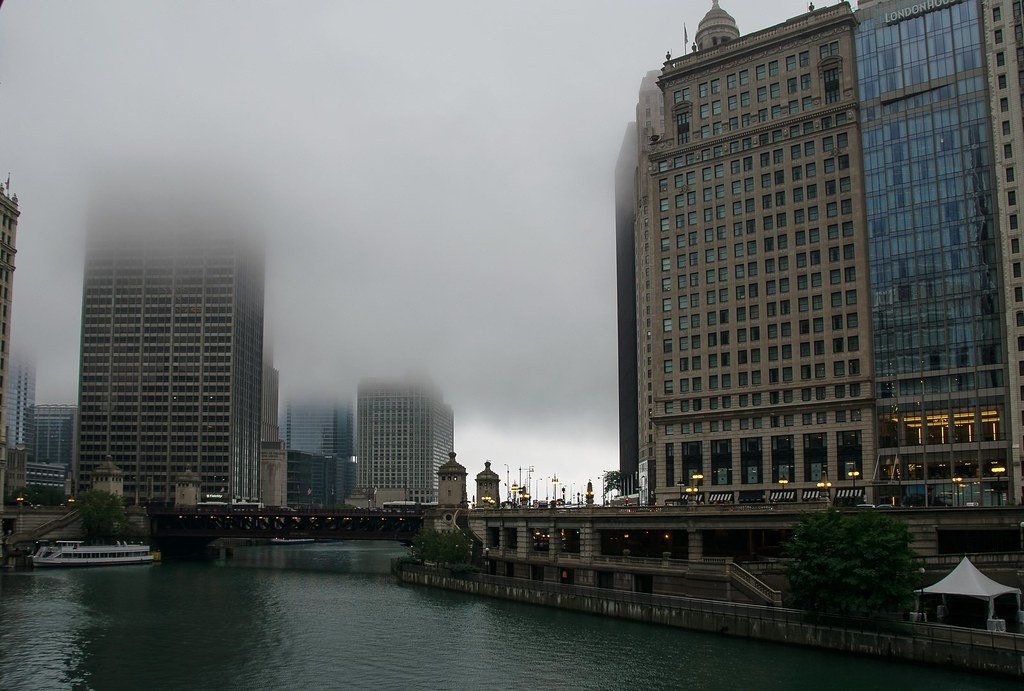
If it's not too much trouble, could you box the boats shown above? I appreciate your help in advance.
[28,540,154,566]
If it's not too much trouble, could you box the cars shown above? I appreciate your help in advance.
[279,507,298,511]
[22,501,33,508]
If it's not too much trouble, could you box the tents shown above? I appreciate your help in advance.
[914,557,1023,632]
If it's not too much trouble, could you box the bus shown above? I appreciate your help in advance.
[382,501,439,515]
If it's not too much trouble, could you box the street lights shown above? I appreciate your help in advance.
[817,471,832,502]
[597,476,604,506]
[455,545,459,554]
[411,545,414,558]
[779,475,788,501]
[918,565,926,623]
[481,496,495,509]
[536,478,542,502]
[546,476,550,500]
[529,466,535,507]
[991,466,1006,507]
[520,493,530,508]
[16,497,25,515]
[405,482,407,515]
[552,478,558,507]
[685,472,704,505]
[511,483,519,508]
[952,474,962,507]
[504,463,510,502]
[485,547,489,573]
[296,484,300,511]
[213,475,216,493]
[848,466,859,508]
[586,492,594,507]
[571,482,576,503]
[677,481,684,502]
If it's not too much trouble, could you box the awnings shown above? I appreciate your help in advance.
[681,493,733,502]
[770,489,863,500]
